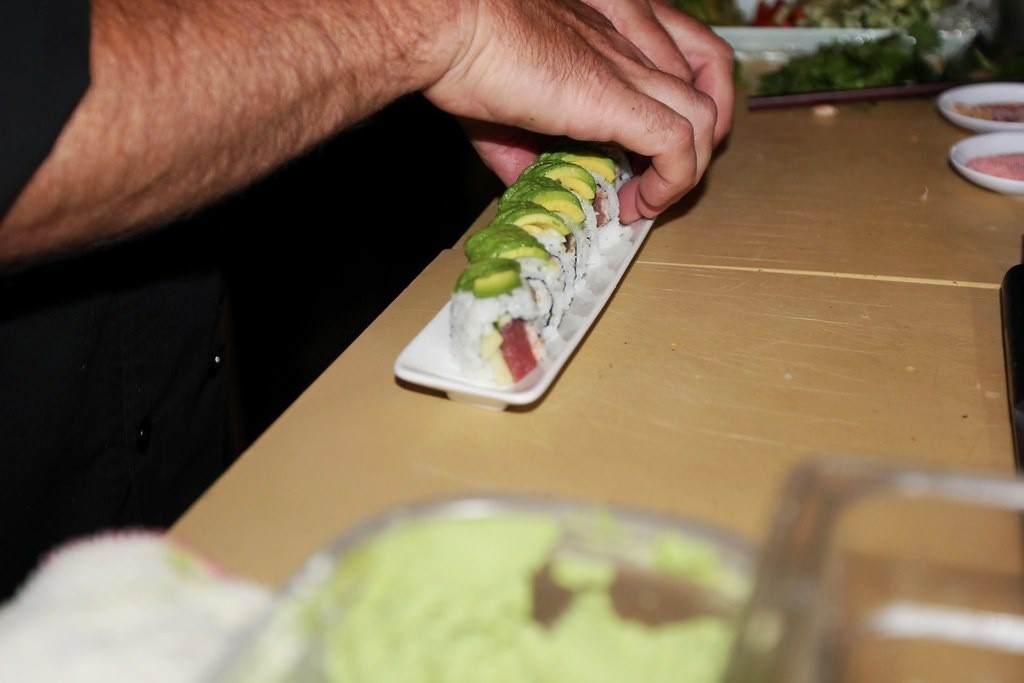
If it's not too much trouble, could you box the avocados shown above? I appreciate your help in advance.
[453,151,616,297]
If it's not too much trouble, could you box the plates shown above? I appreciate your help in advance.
[710,24,888,64]
[394,197,658,411]
[936,82,1024,133]
[948,131,1024,195]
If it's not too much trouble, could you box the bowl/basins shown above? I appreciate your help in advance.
[211,487,854,683]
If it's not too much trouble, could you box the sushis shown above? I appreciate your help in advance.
[449,166,635,361]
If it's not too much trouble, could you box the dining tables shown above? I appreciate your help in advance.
[161,58,1024,683]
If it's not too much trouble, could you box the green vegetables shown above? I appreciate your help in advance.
[755,24,946,94]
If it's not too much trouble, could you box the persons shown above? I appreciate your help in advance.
[0,0,736,608]
